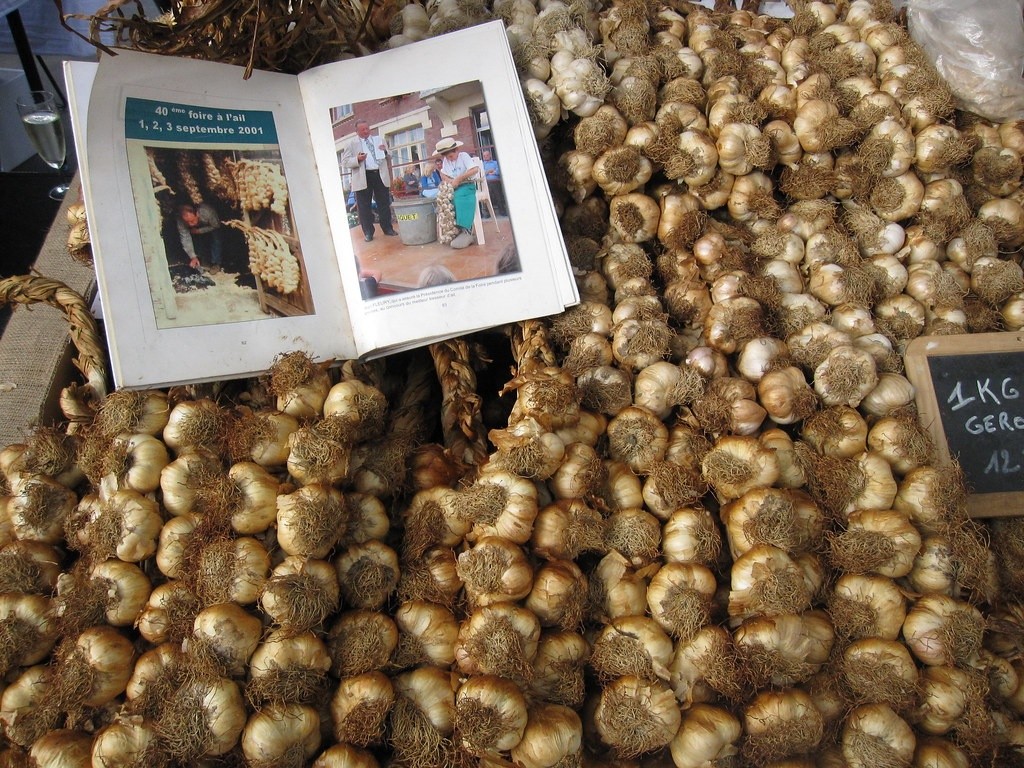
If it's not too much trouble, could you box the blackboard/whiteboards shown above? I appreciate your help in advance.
[906,332,1024,521]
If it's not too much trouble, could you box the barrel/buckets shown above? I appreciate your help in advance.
[391,198,438,246]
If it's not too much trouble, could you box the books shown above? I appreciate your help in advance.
[57,18,583,393]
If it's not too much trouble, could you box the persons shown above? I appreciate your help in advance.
[343,118,522,299]
[177,204,223,276]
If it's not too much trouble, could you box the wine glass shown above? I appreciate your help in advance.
[15,90,72,201]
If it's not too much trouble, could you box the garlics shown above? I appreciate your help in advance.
[0,0,1024,768]
